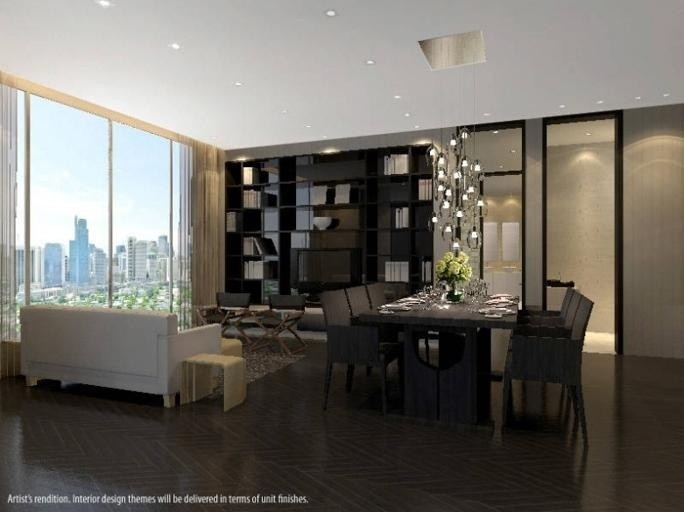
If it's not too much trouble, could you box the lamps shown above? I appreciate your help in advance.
[417,27,486,251]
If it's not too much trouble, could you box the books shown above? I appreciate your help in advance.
[310,182,351,206]
[383,151,433,284]
[225,160,277,299]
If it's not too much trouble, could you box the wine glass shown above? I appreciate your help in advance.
[465,278,488,311]
[417,283,441,310]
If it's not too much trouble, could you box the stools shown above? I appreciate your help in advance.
[180,352,248,414]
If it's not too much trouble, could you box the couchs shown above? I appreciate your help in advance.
[20,305,243,409]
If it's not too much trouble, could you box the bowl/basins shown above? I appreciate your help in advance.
[313,216,332,230]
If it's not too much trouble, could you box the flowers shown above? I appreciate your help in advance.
[435,251,472,286]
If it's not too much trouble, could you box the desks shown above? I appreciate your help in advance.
[297,307,327,332]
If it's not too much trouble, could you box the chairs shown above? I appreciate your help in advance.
[195,292,307,356]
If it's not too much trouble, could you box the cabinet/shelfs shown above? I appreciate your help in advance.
[224,143,434,308]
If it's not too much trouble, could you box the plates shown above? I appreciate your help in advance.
[378,297,424,310]
[379,311,394,314]
[480,293,516,314]
[485,315,501,318]
